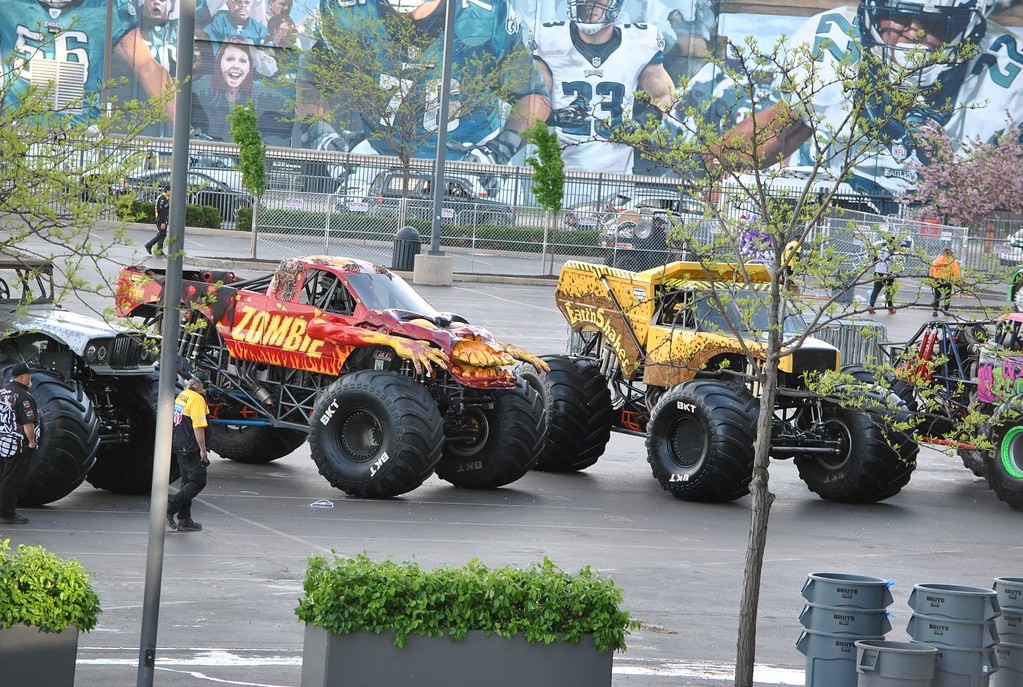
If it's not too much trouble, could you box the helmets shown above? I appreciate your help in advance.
[567,0,624,36]
[858,0,987,94]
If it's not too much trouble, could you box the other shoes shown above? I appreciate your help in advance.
[0,513,29,524]
[944,312,950,315]
[933,312,938,316]
[144,244,152,254]
[160,252,164,255]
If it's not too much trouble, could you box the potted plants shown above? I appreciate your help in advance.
[292,549,643,687]
[0,533,103,687]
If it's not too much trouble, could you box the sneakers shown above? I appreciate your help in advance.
[869,307,876,313]
[166,504,177,530]
[889,309,896,314]
[177,519,203,530]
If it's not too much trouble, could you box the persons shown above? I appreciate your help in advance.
[423,181,431,193]
[931,248,965,316]
[167,371,211,531]
[295,0,1023,214]
[0,0,305,142]
[781,235,802,289]
[867,236,896,314]
[144,186,170,258]
[446,183,454,195]
[0,362,37,524]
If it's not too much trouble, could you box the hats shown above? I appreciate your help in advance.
[189,369,212,383]
[12,364,36,376]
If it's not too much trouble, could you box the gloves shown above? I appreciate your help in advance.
[460,143,510,198]
[608,120,641,146]
[314,132,355,183]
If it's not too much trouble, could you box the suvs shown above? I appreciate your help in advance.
[838,308,1023,516]
[361,165,518,229]
[115,254,552,499]
[0,242,180,507]
[531,260,919,505]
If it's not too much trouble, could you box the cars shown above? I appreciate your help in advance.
[562,185,739,250]
[63,170,128,205]
[109,169,253,224]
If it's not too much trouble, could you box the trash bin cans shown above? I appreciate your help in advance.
[391,227,422,271]
[988,641,1023,687]
[795,627,886,687]
[910,639,999,687]
[992,577,1023,609]
[908,583,1002,621]
[798,602,892,636]
[855,639,939,687]
[906,612,1000,648]
[831,269,857,304]
[994,607,1022,646]
[801,573,894,609]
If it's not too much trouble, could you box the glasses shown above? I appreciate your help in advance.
[202,382,209,389]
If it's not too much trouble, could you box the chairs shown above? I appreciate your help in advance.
[945,324,989,378]
